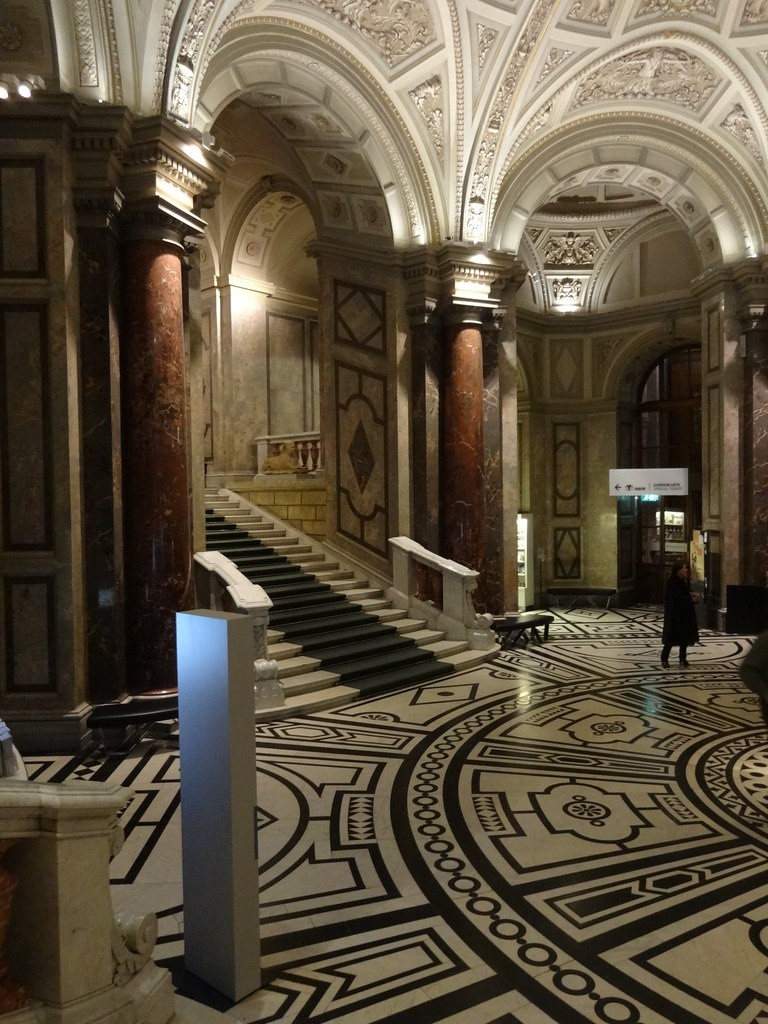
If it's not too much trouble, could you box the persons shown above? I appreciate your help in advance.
[661,560,699,669]
[559,237,577,264]
[625,46,688,91]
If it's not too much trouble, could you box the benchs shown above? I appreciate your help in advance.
[492,614,555,647]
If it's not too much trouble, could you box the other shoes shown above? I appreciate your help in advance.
[661,659,671,669]
[680,657,689,666]
